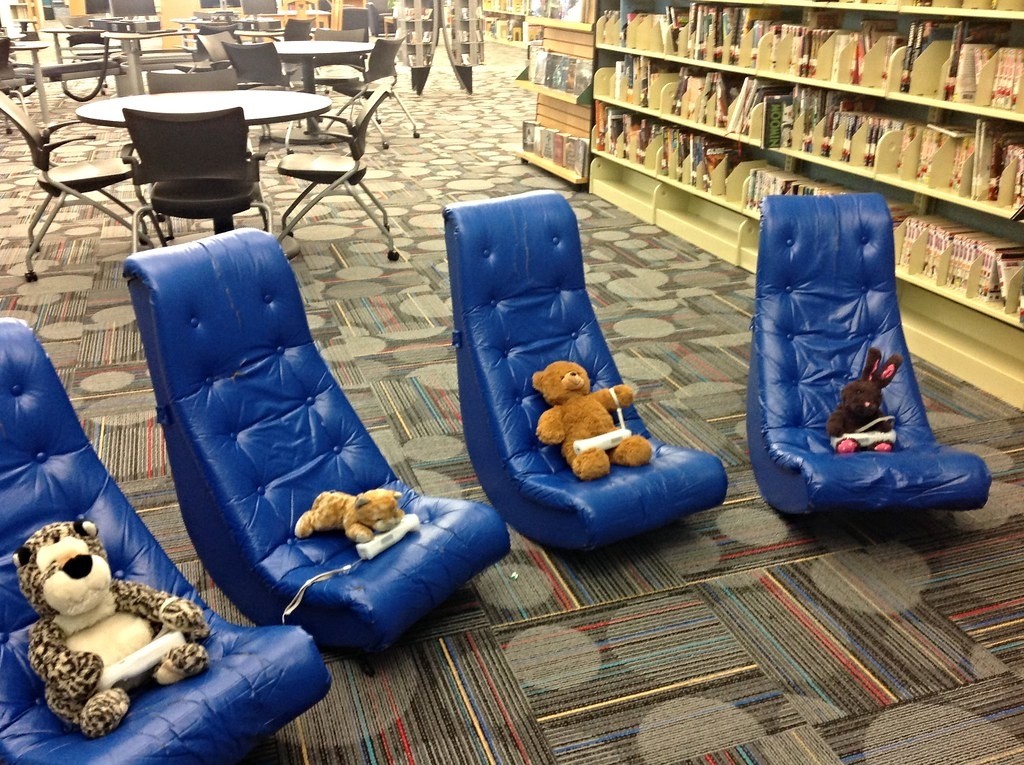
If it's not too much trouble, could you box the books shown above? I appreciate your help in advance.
[397,0,521,67]
[521,1,1024,326]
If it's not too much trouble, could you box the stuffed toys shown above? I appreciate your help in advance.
[294,487,407,545]
[11,520,214,740]
[531,360,653,482]
[825,346,904,455]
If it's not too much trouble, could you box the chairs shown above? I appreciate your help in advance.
[0,1,429,286]
[118,228,512,654]
[744,191,991,519]
[442,189,727,555]
[1,316,333,765]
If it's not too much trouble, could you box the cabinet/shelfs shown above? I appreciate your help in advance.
[520,18,599,185]
[584,1,1023,415]
[481,1,530,48]
